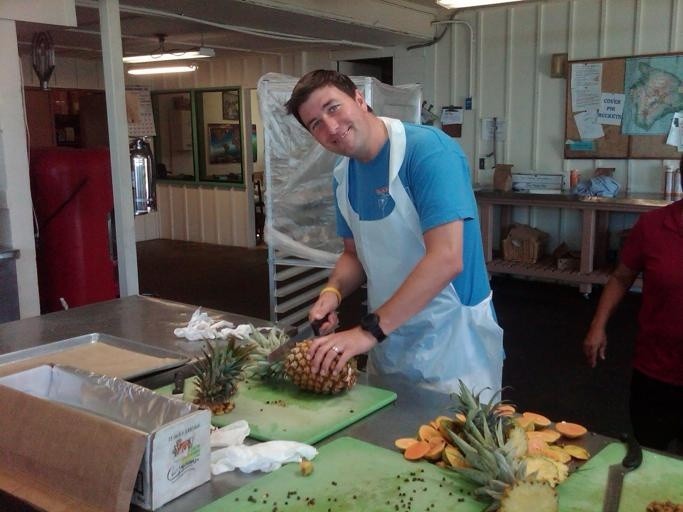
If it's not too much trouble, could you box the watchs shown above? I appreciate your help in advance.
[359,310,385,343]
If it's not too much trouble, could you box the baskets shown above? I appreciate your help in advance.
[503,240,547,264]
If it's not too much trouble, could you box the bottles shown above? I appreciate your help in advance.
[674,169,682,195]
[663,165,673,196]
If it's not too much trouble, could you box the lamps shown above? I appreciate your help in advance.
[122,34,215,76]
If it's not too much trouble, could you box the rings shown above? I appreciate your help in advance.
[330,345,339,354]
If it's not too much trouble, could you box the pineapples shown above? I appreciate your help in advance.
[447,375,561,512]
[646,499,682,511]
[248,329,357,396]
[190,337,256,416]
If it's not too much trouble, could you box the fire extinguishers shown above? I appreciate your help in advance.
[128,135,157,216]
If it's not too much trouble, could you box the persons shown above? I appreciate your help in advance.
[284,68,511,402]
[581,156,682,452]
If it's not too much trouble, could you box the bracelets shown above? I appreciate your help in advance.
[317,287,341,307]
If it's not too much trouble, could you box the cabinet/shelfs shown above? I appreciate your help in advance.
[474,185,683,300]
